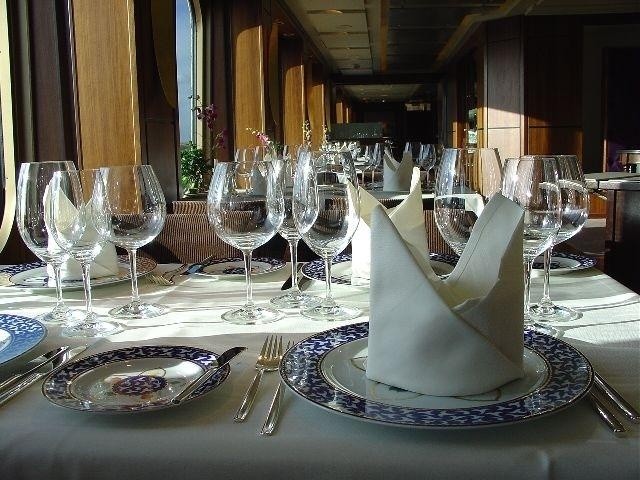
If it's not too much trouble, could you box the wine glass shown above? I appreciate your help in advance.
[291,150,364,322]
[48,166,126,339]
[503,158,561,342]
[407,142,436,191]
[233,146,298,194]
[514,153,590,322]
[16,161,85,328]
[433,147,505,256]
[265,158,322,315]
[352,141,386,190]
[205,160,289,325]
[92,163,173,319]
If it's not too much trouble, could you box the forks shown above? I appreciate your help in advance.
[147,264,189,286]
[259,338,298,437]
[232,333,285,423]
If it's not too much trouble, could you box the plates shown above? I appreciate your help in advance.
[279,321,593,430]
[189,254,287,278]
[529,247,596,276]
[44,346,233,413]
[1,254,157,290]
[0,312,46,363]
[301,252,464,285]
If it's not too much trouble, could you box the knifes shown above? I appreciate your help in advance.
[170,344,247,406]
[1,344,89,406]
[1,344,72,388]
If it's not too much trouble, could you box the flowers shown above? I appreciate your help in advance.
[321,122,329,144]
[244,125,283,160]
[302,119,312,148]
[178,94,231,194]
